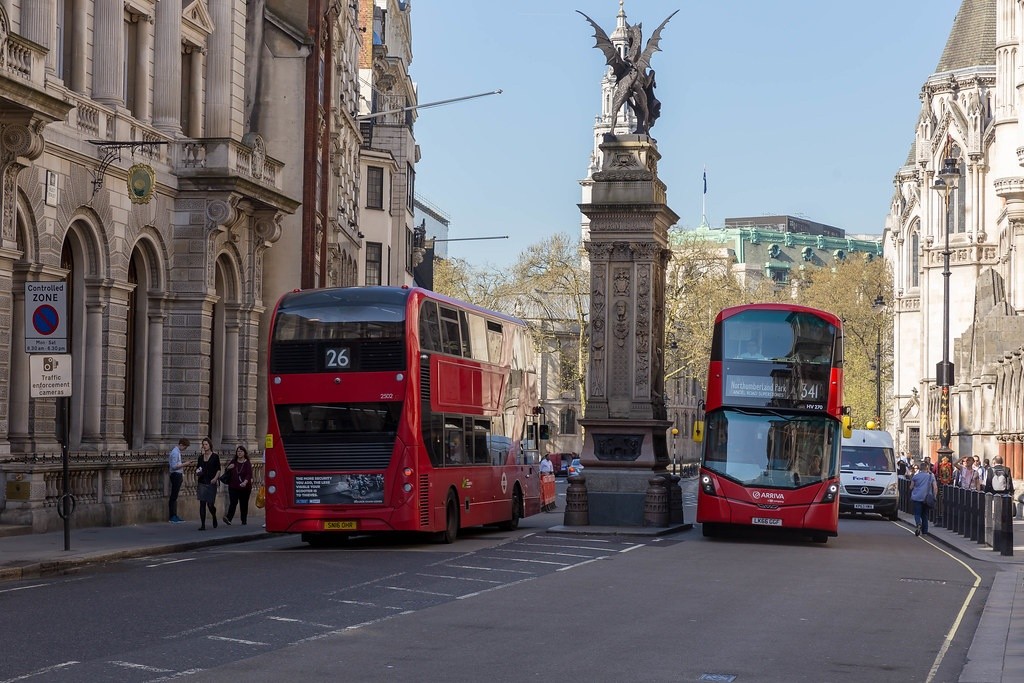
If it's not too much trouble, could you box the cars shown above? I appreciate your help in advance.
[568,459,585,483]
[453,435,524,466]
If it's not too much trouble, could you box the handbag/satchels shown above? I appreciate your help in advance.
[924,494,936,508]
[220,470,232,484]
[255,485,266,508]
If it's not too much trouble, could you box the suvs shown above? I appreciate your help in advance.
[540,452,581,477]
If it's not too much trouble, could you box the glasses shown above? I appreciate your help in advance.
[974,460,979,462]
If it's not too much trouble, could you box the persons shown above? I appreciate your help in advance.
[910,463,938,537]
[168,438,190,523]
[896,452,914,474]
[808,455,827,476]
[222,446,253,525]
[742,340,764,358]
[925,457,934,471]
[195,438,221,531]
[540,454,553,474]
[953,455,1014,495]
[261,448,266,527]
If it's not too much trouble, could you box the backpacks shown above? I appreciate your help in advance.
[992,467,1007,491]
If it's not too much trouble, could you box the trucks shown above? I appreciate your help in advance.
[839,429,901,519]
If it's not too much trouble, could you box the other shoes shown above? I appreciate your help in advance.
[923,532,927,536]
[213,517,217,528]
[169,516,181,525]
[177,516,186,522]
[262,523,266,527]
[915,523,922,536]
[223,516,232,525]
[241,521,247,525]
[198,525,205,531]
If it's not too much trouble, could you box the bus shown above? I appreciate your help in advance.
[693,303,854,546]
[256,283,551,541]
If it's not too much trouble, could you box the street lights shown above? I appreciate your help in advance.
[671,428,679,474]
[929,131,965,487]
[873,282,884,429]
